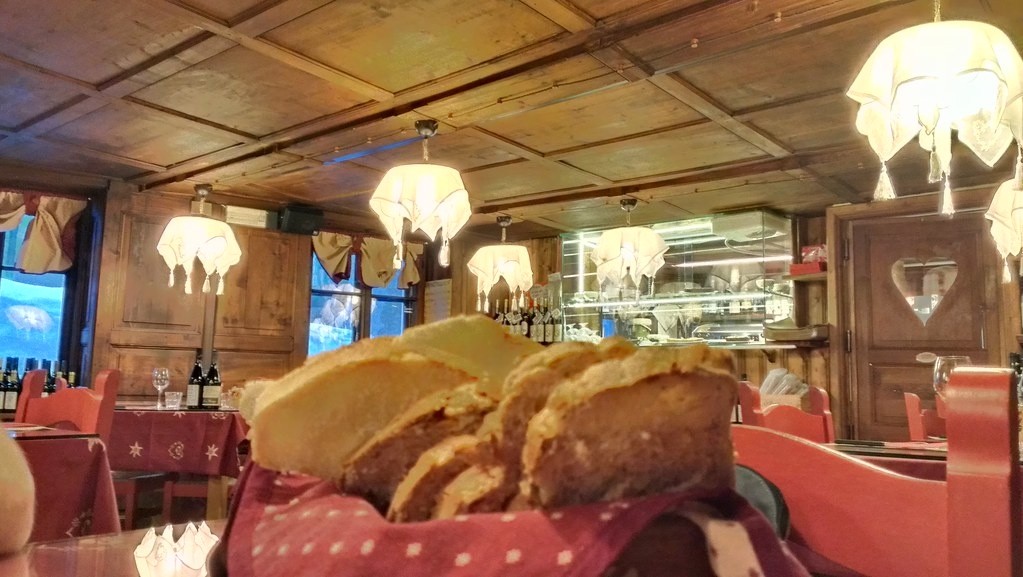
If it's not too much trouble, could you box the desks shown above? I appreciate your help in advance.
[0,515,231,577]
[761,437,949,577]
[53,410,248,521]
[1,420,121,548]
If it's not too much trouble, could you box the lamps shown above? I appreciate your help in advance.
[591,199,670,289]
[370,119,470,270]
[469,216,534,314]
[156,185,242,294]
[846,0,1023,201]
[978,144,1023,284]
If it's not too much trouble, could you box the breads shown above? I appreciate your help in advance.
[239,312,739,520]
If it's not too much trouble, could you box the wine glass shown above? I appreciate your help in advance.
[152,367,170,409]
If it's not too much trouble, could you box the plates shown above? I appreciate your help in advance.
[660,336,752,346]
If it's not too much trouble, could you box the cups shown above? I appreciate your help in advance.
[164,392,182,409]
[220,393,234,410]
[932,355,972,403]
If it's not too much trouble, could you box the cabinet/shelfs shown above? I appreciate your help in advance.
[559,202,803,351]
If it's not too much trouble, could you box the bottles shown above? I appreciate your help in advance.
[493,296,563,345]
[186,348,222,409]
[1009,351,1023,375]
[0,355,75,413]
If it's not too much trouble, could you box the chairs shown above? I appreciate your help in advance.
[735,358,1023,577]
[15,369,245,532]
